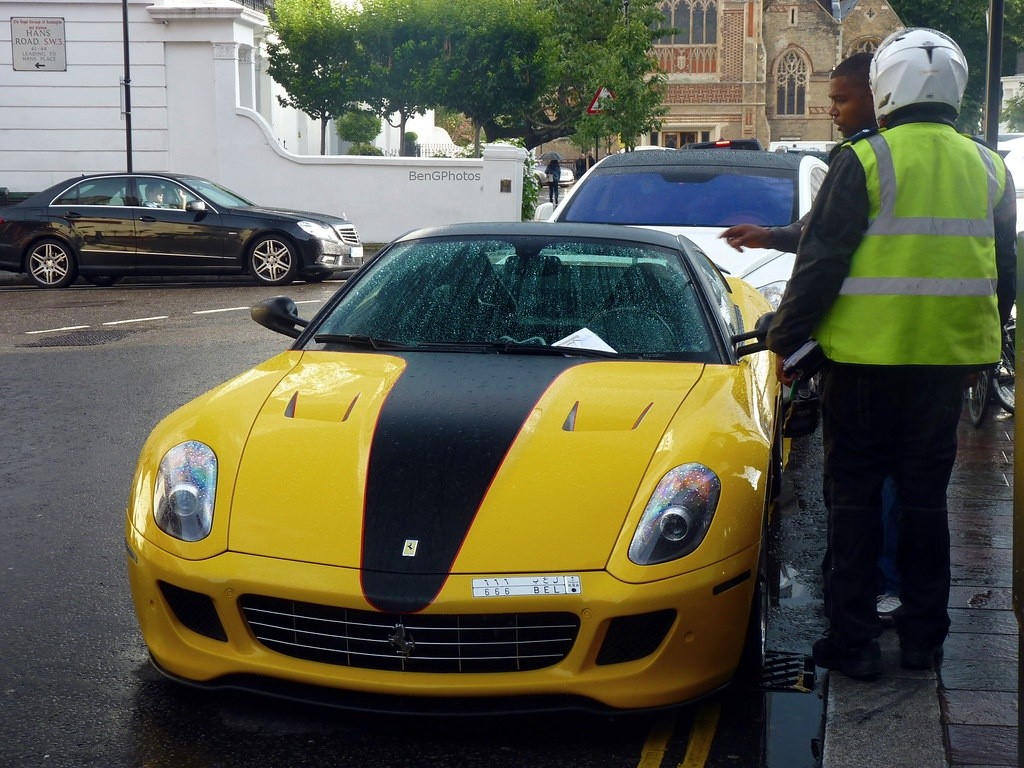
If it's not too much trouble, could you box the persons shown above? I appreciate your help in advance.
[144,184,187,210]
[545,160,560,204]
[574,150,595,180]
[768,27,1016,668]
[719,53,902,620]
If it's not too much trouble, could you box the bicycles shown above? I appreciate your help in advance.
[967,316,1016,428]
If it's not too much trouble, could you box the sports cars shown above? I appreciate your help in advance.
[122,220,813,714]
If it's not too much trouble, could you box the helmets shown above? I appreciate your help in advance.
[869,27,969,128]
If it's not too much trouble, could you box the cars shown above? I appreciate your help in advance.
[532,148,831,405]
[2,171,366,288]
[532,164,575,188]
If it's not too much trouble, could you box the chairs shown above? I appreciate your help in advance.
[78,184,176,206]
[400,248,708,358]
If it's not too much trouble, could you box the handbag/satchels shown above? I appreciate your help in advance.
[546,174,554,183]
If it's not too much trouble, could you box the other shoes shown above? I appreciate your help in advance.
[898,628,948,670]
[875,592,901,616]
[812,628,881,683]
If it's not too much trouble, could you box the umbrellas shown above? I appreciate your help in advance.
[537,151,565,160]
